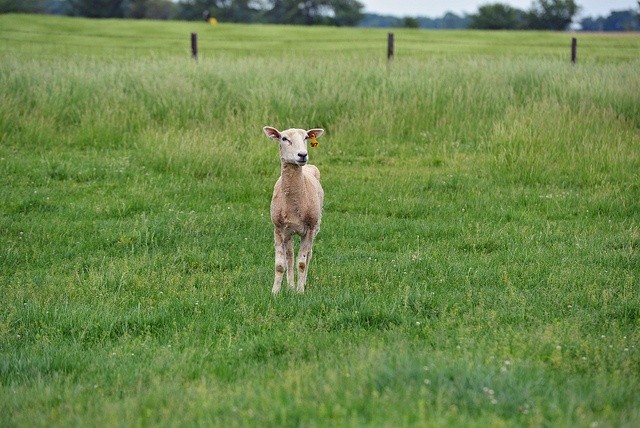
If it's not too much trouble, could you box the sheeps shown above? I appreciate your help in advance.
[263,124,325,298]
